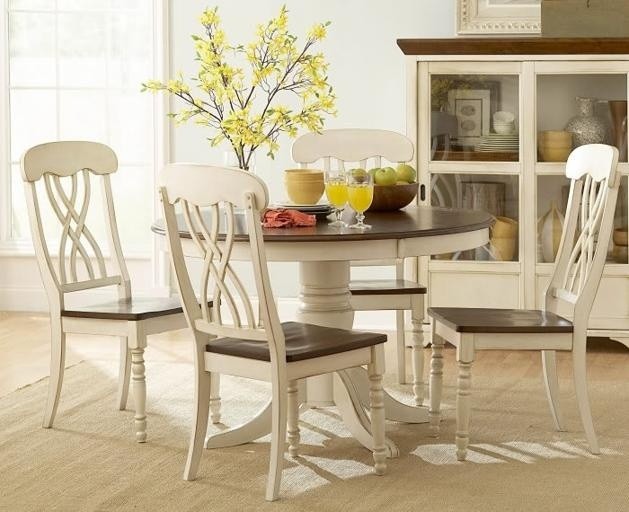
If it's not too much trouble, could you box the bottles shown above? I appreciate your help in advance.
[537,199,565,263]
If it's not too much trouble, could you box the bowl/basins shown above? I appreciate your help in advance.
[492,110,515,134]
[612,228,628,264]
[364,181,420,212]
[283,169,324,205]
[539,130,572,162]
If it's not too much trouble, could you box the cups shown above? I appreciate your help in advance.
[486,213,518,261]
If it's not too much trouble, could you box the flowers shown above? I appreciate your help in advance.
[143,3,340,171]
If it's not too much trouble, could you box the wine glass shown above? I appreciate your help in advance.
[323,171,348,228]
[347,175,375,229]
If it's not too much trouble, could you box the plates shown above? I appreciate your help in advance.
[474,132,519,153]
[274,203,334,219]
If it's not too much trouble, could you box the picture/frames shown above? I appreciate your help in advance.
[437,79,503,152]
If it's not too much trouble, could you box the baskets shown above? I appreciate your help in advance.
[298,208,331,219]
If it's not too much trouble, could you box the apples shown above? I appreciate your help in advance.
[350,163,416,188]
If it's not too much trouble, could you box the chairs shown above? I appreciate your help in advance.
[22,131,618,501]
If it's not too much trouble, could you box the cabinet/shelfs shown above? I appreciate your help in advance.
[396,35,629,350]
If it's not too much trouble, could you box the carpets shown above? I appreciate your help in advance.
[0,327,629,512]
